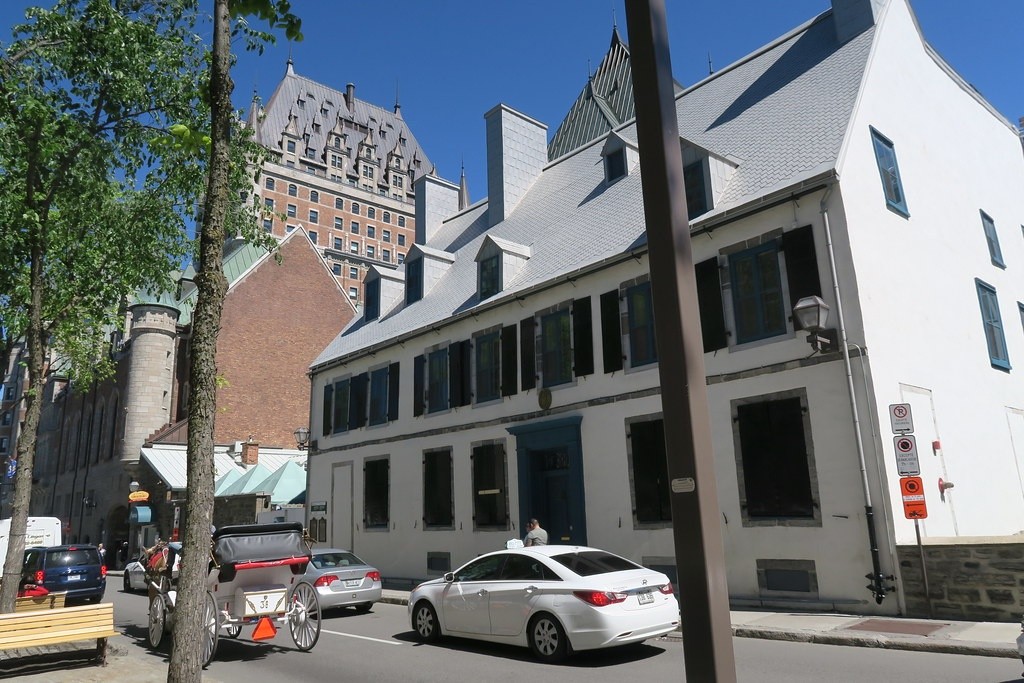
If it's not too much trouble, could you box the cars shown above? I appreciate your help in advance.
[408,543,680,664]
[18,543,107,607]
[122,541,183,593]
[301,547,382,617]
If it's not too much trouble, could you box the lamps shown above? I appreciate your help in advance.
[83,497,97,508]
[793,296,839,354]
[294,426,318,452]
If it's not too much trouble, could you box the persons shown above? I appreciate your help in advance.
[523,518,548,546]
[98,543,106,561]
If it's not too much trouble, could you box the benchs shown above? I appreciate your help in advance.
[16,593,67,612]
[210,557,309,590]
[0,602,120,668]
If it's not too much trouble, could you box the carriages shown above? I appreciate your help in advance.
[139,518,322,670]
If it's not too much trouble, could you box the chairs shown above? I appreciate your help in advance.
[338,559,351,567]
